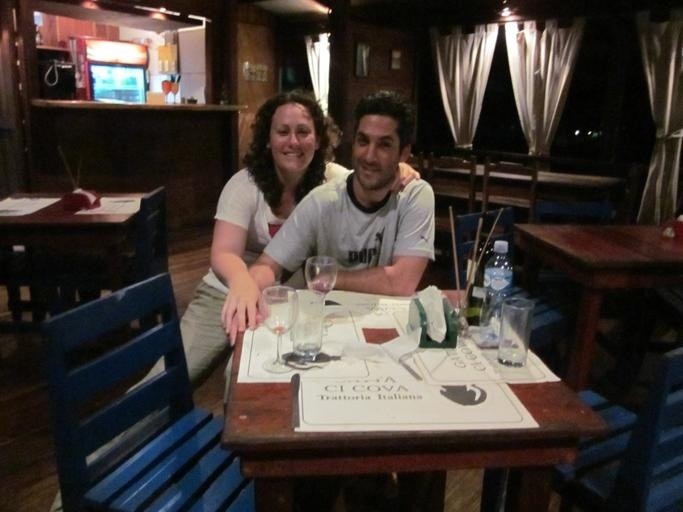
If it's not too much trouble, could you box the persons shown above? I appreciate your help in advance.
[222,90,435,511]
[50,91,421,512]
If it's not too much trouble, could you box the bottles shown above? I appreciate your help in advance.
[464,211,494,326]
[477,239,513,347]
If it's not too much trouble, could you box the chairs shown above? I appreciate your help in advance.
[409,149,539,279]
[526,345,682,511]
[447,209,572,368]
[83,188,163,336]
[42,270,256,510]
[528,189,634,319]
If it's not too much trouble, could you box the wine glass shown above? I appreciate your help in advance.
[303,256,339,327]
[258,285,297,374]
[162,81,178,104]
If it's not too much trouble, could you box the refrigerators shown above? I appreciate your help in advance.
[66,34,149,104]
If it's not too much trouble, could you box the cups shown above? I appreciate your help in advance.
[287,289,324,356]
[494,297,534,367]
